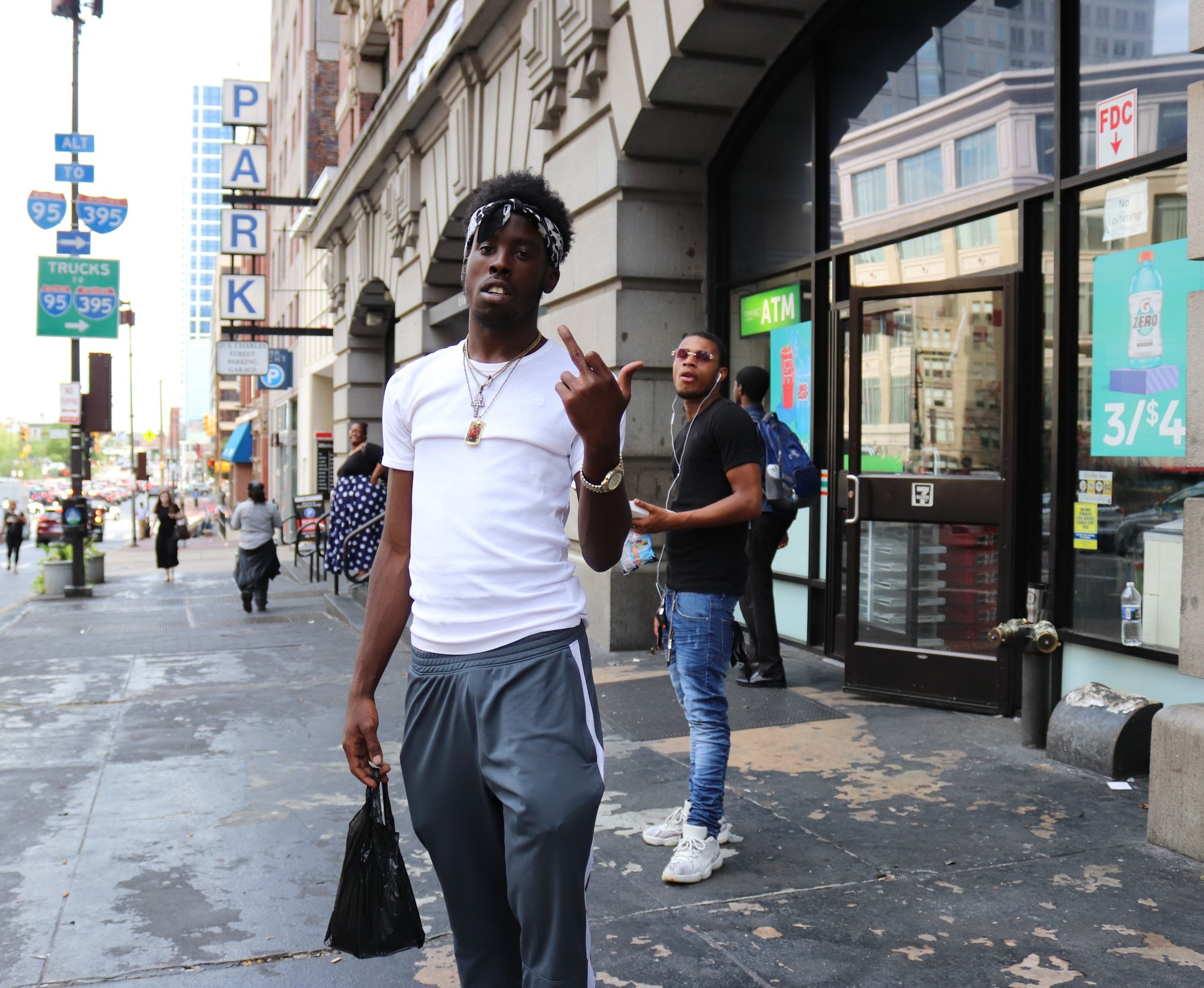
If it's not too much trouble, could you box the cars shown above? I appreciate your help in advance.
[27,476,166,506]
[183,482,213,498]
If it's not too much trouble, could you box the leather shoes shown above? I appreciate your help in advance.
[735,671,786,688]
[736,660,759,673]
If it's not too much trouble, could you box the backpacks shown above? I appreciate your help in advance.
[749,409,821,511]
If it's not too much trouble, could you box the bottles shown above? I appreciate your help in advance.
[1120,581,1143,647]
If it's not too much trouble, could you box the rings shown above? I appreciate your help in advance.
[631,524,635,528]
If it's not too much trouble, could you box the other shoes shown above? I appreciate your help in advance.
[347,576,368,588]
[256,605,265,611]
[242,592,253,612]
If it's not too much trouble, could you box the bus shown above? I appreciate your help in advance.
[190,471,206,483]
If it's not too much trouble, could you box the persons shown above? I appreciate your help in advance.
[629,331,764,884]
[342,171,644,988]
[1,501,27,571]
[191,487,198,507]
[338,421,386,589]
[734,366,797,689]
[230,482,282,613]
[149,491,182,582]
[135,503,149,540]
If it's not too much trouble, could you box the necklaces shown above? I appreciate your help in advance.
[462,332,542,445]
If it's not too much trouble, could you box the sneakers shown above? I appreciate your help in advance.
[641,799,733,843]
[660,822,724,885]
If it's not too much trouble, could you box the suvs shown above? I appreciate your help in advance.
[36,508,66,545]
[1115,481,1204,556]
[1083,500,1125,553]
[81,497,110,542]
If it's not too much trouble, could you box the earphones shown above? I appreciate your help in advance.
[716,373,722,383]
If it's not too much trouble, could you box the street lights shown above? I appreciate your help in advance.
[119,300,137,548]
[159,380,164,493]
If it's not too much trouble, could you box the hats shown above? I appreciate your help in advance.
[248,480,264,491]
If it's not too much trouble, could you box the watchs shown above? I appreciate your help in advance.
[580,454,625,493]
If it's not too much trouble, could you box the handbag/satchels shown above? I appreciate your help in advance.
[323,770,426,960]
[177,525,190,538]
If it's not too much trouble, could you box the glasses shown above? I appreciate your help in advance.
[671,348,720,366]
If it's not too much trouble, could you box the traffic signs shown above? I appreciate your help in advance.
[36,256,119,338]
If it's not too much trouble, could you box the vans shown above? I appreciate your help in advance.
[0,476,31,543]
